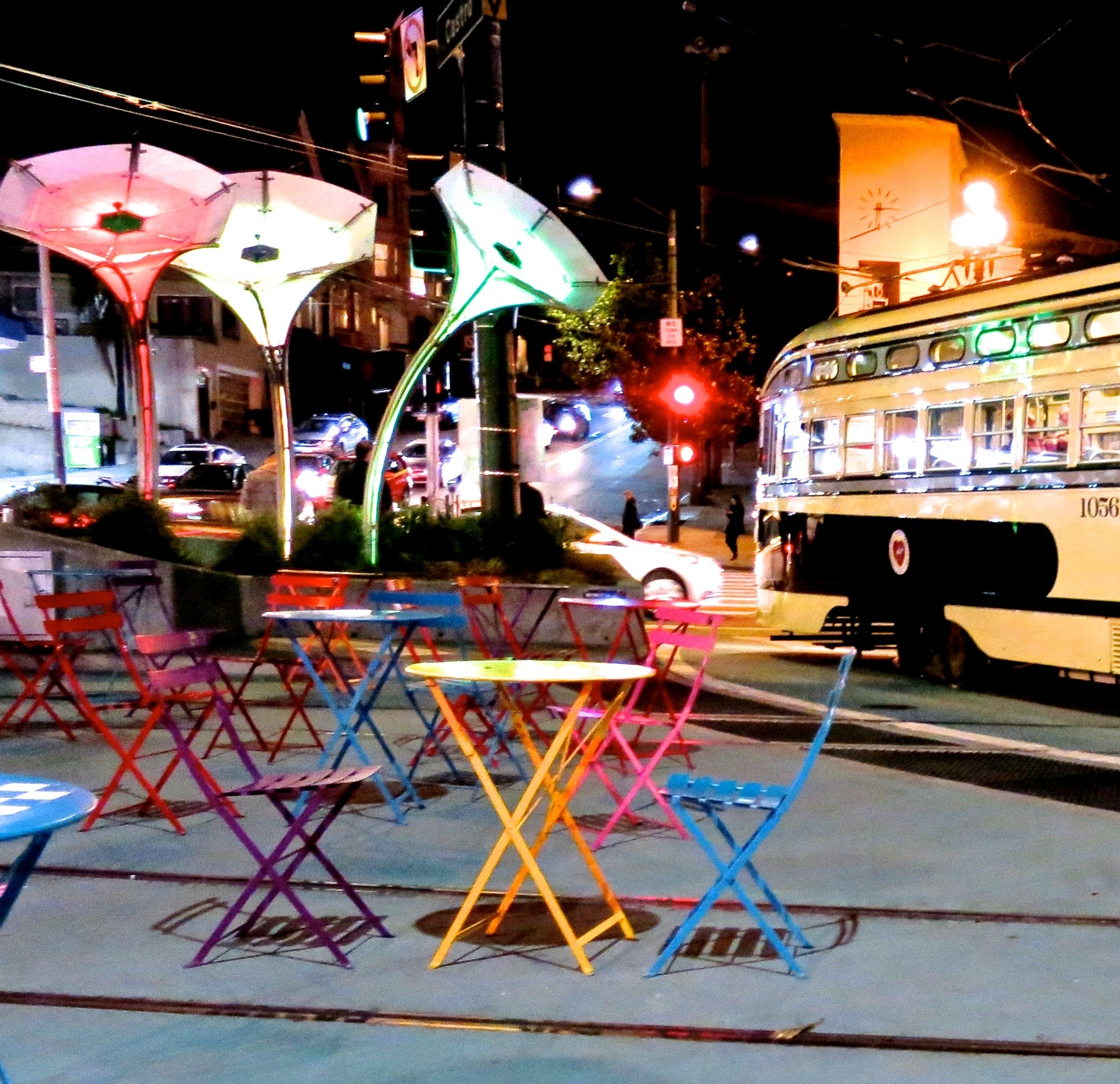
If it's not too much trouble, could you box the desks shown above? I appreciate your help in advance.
[263,611,440,831]
[0,772,99,924]
[553,595,701,768]
[401,660,654,965]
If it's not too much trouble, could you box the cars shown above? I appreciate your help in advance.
[541,398,592,450]
[1,470,176,657]
[0,395,459,538]
[460,498,725,622]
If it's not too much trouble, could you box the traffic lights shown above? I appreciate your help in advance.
[669,380,703,410]
[404,151,461,277]
[352,27,402,145]
[674,443,699,464]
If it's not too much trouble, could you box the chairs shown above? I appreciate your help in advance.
[0,557,857,958]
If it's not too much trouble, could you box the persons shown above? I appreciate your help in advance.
[240,451,305,517]
[724,495,746,560]
[1002,405,1090,457]
[336,441,393,513]
[621,490,643,539]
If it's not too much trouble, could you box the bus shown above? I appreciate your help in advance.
[751,235,1120,703]
